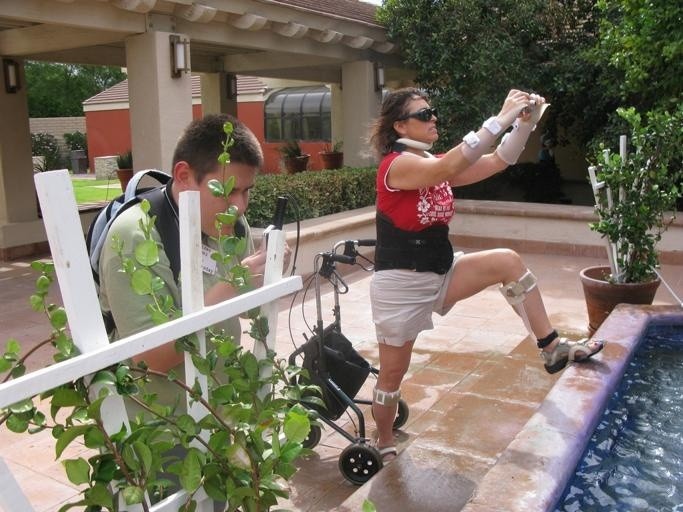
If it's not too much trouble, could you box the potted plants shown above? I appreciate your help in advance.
[32,159,50,219]
[579,106,683,338]
[115,150,135,194]
[274,136,311,174]
[317,136,345,170]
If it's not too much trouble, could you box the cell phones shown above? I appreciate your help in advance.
[261,225,275,251]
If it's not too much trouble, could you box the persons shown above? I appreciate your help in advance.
[86,113,294,511]
[368,86,607,468]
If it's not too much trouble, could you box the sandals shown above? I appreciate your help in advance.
[372,440,397,466]
[541,337,603,374]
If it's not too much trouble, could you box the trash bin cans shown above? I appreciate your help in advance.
[69,149,87,174]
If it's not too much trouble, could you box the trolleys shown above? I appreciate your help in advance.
[281,237,412,486]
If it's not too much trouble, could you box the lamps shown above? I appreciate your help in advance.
[2,57,22,94]
[168,35,190,79]
[225,74,237,101]
[373,64,386,91]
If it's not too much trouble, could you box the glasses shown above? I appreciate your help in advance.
[396,107,439,123]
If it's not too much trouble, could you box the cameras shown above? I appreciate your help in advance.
[519,96,536,115]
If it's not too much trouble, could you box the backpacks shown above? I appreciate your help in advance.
[86,167,183,341]
